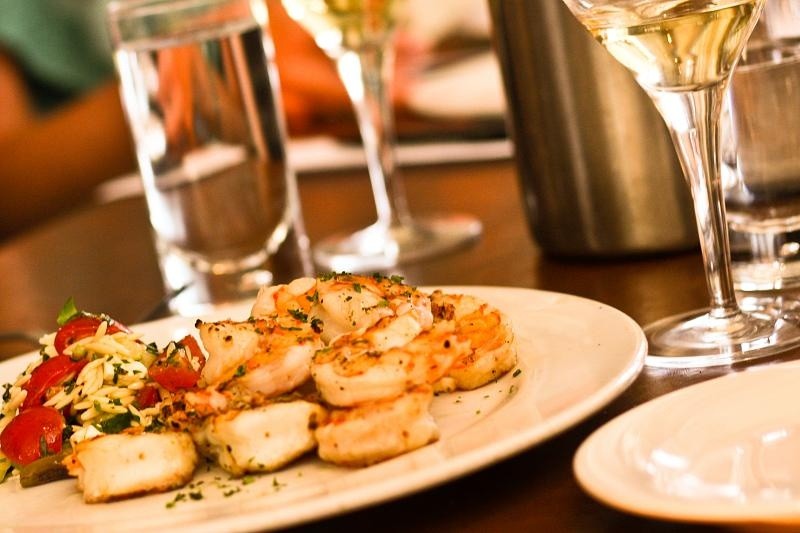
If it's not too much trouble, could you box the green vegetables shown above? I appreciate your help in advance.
[0,271,421,510]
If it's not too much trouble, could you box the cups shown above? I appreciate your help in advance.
[719,0,800,295]
[486,0,702,261]
[104,0,313,312]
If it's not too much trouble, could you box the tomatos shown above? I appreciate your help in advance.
[54,312,129,353]
[18,354,88,413]
[0,406,65,468]
[149,335,206,392]
[134,385,159,408]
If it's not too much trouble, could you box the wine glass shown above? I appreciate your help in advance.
[274,0,488,276]
[556,0,800,369]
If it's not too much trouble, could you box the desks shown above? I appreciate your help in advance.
[1,116,800,533]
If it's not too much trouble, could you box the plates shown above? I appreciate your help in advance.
[570,358,800,533]
[0,285,649,533]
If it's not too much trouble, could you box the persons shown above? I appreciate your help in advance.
[0,0,358,242]
